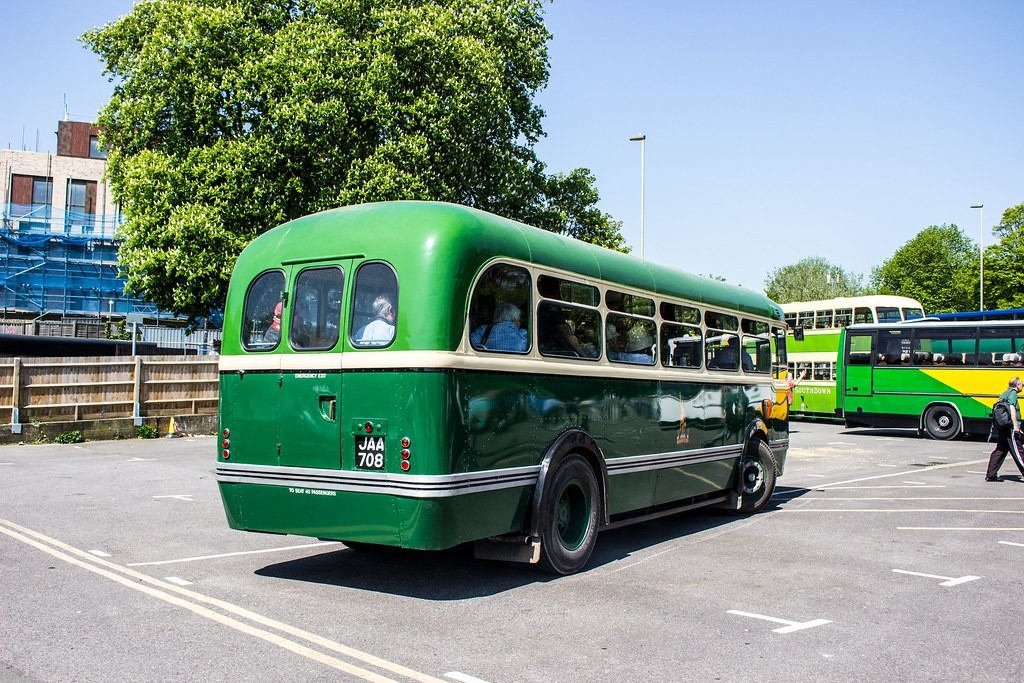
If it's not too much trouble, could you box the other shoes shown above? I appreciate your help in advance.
[985,477,1004,482]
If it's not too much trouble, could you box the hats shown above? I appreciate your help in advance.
[605,323,620,341]
[625,326,654,352]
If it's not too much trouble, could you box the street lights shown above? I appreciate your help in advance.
[970,203,985,313]
[630,134,646,257]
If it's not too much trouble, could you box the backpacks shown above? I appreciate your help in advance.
[992,389,1018,430]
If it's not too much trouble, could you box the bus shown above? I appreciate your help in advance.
[834,306,1022,441]
[776,294,927,428]
[214,201,806,577]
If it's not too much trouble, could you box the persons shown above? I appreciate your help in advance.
[788,370,805,408]
[901,356,910,365]
[985,377,1024,482]
[879,354,892,364]
[540,305,654,364]
[711,337,755,370]
[264,303,310,350]
[933,356,946,366]
[470,303,528,353]
[837,321,843,327]
[826,322,831,328]
[1009,357,1023,366]
[352,292,396,347]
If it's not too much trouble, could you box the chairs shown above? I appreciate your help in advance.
[859,348,1024,368]
[247,340,721,370]
